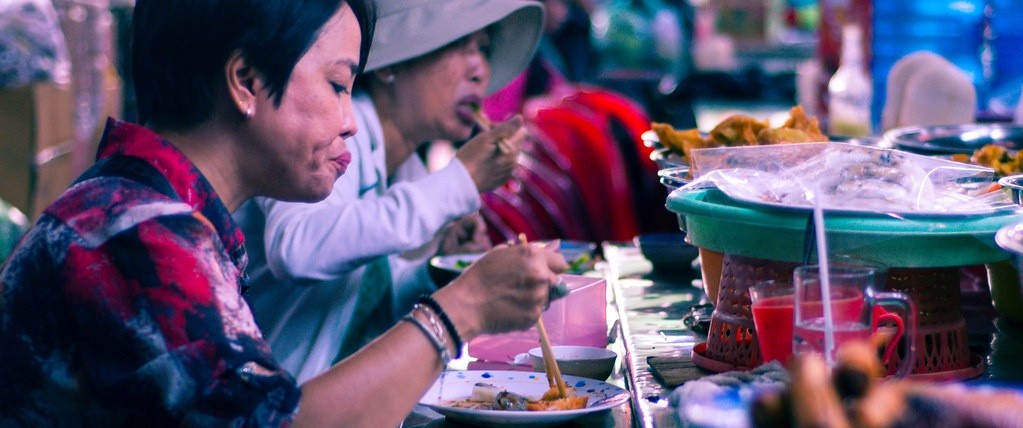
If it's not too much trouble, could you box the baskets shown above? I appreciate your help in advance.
[691,251,984,381]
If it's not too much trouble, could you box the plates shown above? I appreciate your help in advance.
[419,370,632,425]
[718,180,994,214]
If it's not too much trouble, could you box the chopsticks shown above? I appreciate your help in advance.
[509,233,567,399]
[473,112,517,153]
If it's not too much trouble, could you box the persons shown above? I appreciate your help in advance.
[0,0,1023,428]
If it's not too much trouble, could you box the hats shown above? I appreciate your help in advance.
[361,0,545,98]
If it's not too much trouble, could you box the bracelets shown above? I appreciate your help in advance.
[410,304,451,400]
[400,314,446,365]
[416,294,463,359]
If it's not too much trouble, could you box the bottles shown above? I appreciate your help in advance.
[828,22,870,139]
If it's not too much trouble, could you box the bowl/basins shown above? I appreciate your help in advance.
[885,124,1023,156]
[635,232,700,266]
[429,251,484,290]
[535,240,595,261]
[529,344,617,381]
[998,174,1023,205]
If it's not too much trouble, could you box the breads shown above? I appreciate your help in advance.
[527,395,589,412]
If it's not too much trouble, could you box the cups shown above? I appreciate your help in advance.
[749,257,918,380]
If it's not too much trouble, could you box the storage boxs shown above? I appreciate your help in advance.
[468,275,607,364]
